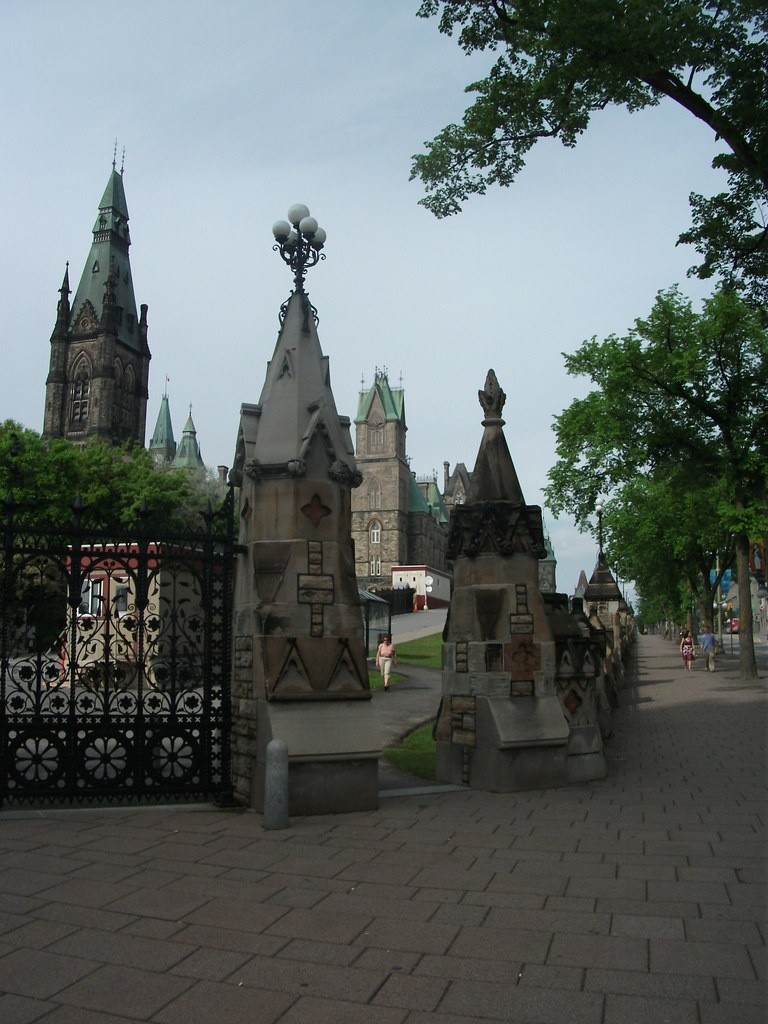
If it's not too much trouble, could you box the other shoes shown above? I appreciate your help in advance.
[705,667,708,671]
[685,666,687,669]
[688,669,692,671]
[711,671,714,673]
[384,686,389,691]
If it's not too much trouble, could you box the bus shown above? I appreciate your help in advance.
[726,618,738,634]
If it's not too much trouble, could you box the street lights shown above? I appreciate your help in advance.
[615,561,618,586]
[625,588,627,604]
[622,579,624,600]
[595,498,606,564]
[628,594,629,607]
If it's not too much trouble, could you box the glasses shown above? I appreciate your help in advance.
[384,640,388,641]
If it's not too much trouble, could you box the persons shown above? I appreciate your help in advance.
[700,627,718,673]
[375,635,397,692]
[679,631,696,671]
[644,627,647,634]
